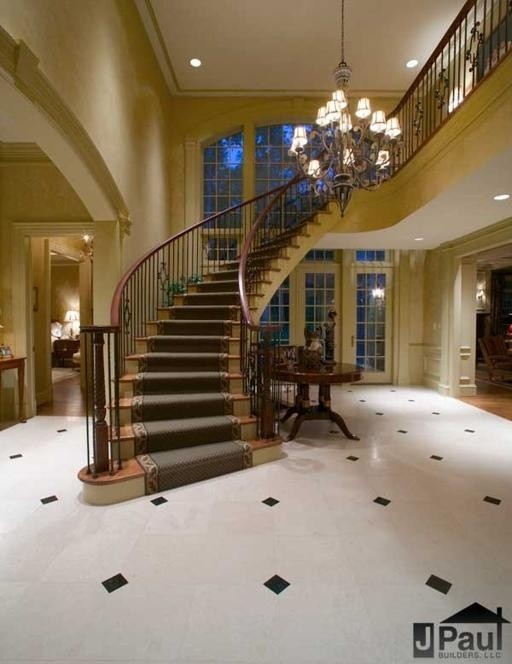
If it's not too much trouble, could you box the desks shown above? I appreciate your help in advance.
[259,358,364,445]
[0,352,32,426]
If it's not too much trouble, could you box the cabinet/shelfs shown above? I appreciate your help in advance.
[51,339,80,366]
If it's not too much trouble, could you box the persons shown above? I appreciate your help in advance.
[485,39,512,75]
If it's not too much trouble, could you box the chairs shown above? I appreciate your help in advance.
[72,348,82,370]
[477,335,512,387]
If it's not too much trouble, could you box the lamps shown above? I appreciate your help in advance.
[284,0,402,221]
[370,283,385,307]
[64,309,79,338]
